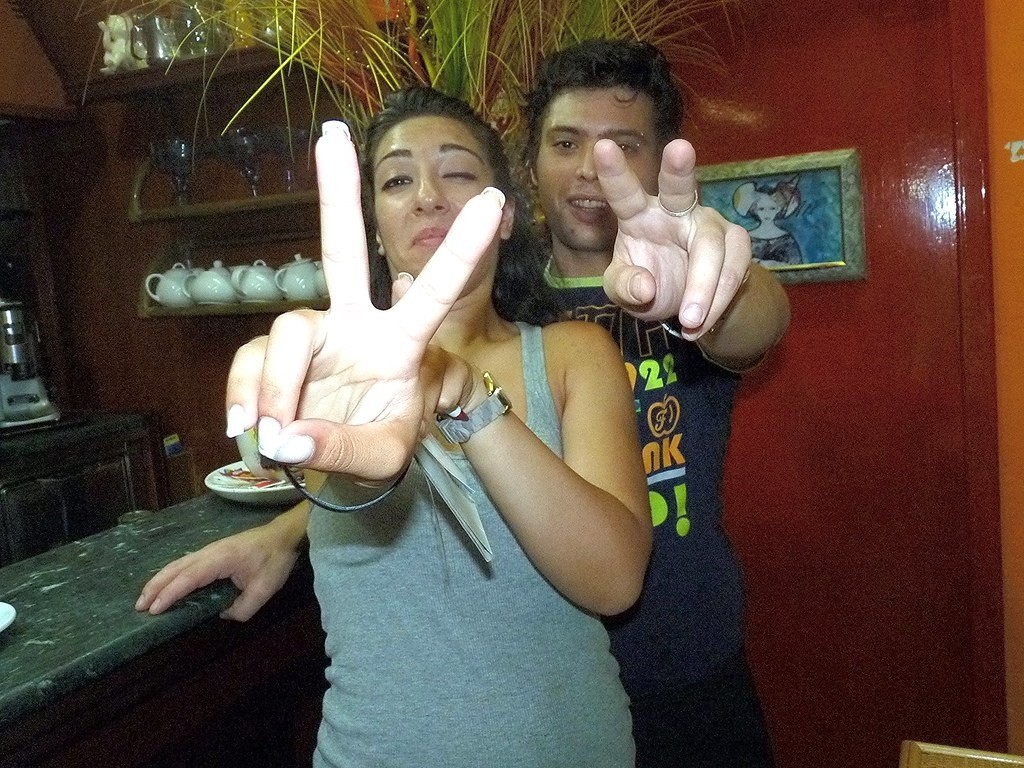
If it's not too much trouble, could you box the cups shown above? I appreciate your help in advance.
[230,259,283,304]
[275,254,318,301]
[130,17,182,64]
[174,0,218,59]
[312,261,330,299]
[144,263,195,307]
[181,260,239,304]
[235,426,288,481]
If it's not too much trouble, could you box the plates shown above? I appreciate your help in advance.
[0,601,16,632]
[204,462,306,503]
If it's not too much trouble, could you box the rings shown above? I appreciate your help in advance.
[658,189,699,217]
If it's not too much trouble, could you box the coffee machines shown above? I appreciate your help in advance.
[0,298,60,427]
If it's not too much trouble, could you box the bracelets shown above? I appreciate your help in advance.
[659,268,751,340]
[284,465,409,513]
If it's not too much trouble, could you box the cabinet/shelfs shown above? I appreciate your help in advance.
[0,420,168,569]
[66,22,380,321]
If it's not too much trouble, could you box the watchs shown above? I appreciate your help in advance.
[434,369,513,445]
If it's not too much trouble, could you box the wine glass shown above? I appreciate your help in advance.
[152,125,301,205]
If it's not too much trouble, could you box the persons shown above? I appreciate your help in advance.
[224,86,653,768]
[136,30,792,768]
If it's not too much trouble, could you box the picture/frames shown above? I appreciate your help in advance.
[693,147,867,285]
